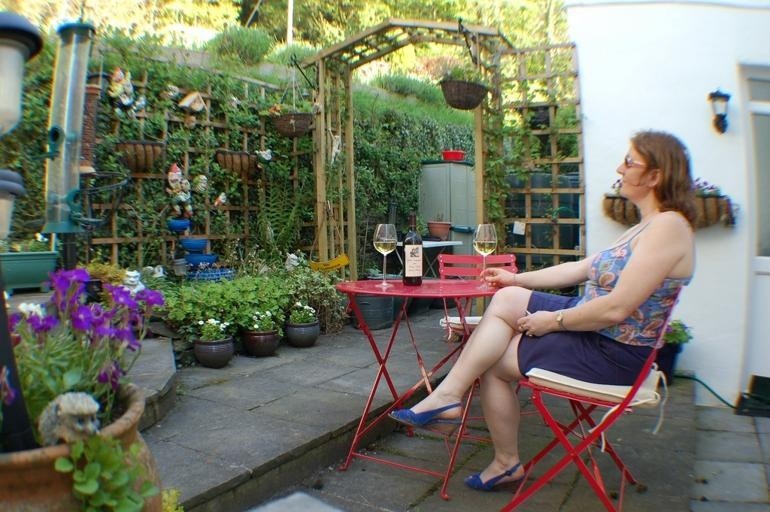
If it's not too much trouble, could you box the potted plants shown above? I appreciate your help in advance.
[267,102,313,140]
[438,61,497,112]
[426,211,452,242]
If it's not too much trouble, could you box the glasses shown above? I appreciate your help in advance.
[624,155,647,169]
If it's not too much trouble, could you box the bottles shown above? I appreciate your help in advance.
[402,214,425,286]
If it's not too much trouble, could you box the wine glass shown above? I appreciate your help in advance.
[473,223,498,290]
[373,223,398,288]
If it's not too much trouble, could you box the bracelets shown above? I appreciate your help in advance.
[512,271,518,285]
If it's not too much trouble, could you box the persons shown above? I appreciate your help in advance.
[385,127,702,491]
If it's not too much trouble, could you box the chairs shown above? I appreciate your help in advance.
[500,287,686,512]
[439,252,517,345]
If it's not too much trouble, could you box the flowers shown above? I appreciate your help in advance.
[0,266,165,403]
[609,175,724,198]
[187,300,318,338]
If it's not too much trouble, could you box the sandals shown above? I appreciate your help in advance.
[463,461,526,494]
[386,402,463,438]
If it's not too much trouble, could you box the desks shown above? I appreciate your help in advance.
[329,276,500,501]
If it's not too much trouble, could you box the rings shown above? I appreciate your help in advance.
[521,323,525,331]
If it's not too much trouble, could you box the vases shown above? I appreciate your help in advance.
[601,195,734,230]
[2,380,165,512]
[191,323,320,369]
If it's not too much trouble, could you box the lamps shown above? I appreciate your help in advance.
[707,86,733,135]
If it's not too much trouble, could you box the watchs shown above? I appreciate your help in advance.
[557,308,568,330]
